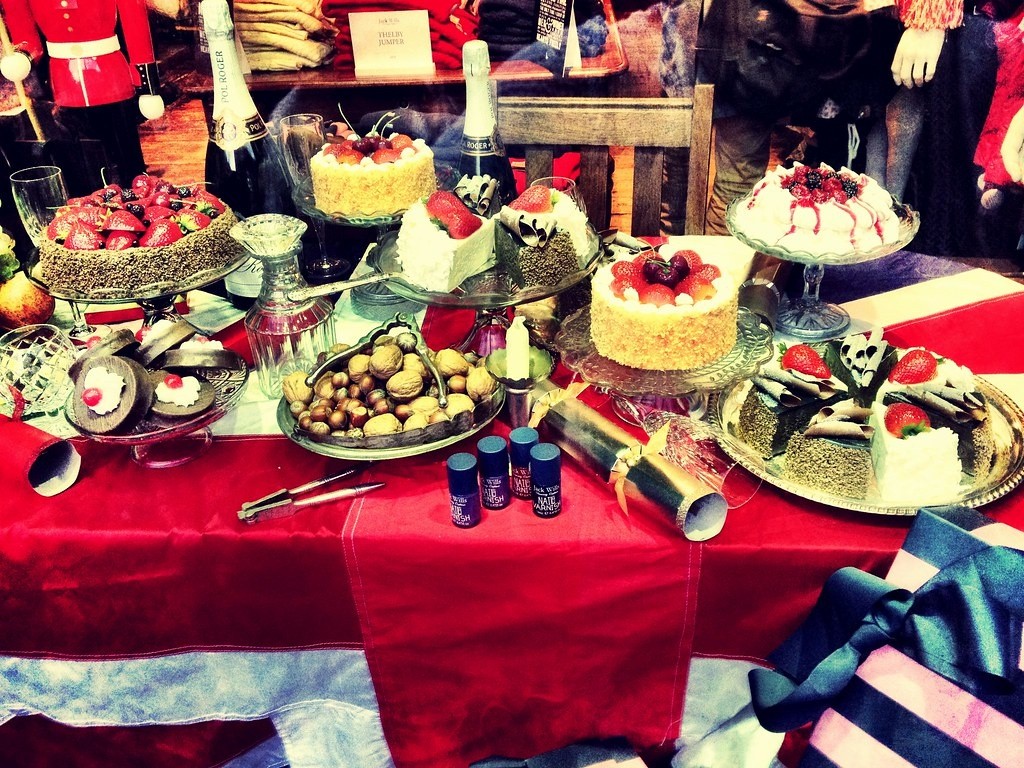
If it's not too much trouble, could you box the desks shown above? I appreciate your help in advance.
[0,225,1024,767]
[175,0,628,203]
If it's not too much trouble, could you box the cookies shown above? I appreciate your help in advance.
[67,320,243,433]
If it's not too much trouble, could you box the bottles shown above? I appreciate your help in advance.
[199,0,300,304]
[455,39,517,215]
[509,427,539,499]
[530,442,562,519]
[476,434,509,510]
[445,452,481,527]
[228,214,340,401]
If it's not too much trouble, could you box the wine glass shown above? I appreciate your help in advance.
[1,324,84,446]
[570,368,767,509]
[10,165,110,353]
[280,112,349,280]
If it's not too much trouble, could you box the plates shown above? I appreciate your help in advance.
[706,363,1024,516]
[271,391,506,460]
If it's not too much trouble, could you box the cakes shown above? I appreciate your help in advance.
[396,186,590,292]
[737,327,993,506]
[591,249,739,371]
[310,132,437,218]
[38,175,245,299]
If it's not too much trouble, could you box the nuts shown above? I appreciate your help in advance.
[284,333,499,437]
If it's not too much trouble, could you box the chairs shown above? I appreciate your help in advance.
[489,79,716,245]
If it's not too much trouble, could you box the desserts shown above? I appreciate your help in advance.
[736,161,900,254]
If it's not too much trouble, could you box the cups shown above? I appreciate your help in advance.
[530,177,587,216]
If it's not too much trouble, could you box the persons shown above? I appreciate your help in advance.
[0,0,165,187]
[862,0,965,200]
